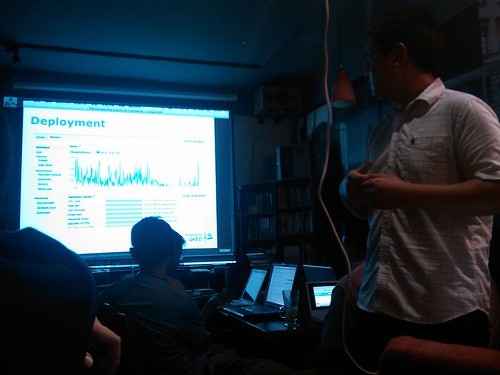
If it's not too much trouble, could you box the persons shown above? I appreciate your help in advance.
[94,218,233,374]
[0,224,95,375]
[316,200,371,373]
[337,6,500,375]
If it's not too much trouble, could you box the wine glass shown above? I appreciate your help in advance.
[282,289,299,329]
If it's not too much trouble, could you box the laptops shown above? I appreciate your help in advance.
[302,264,337,282]
[306,281,339,324]
[225,269,268,307]
[223,262,298,319]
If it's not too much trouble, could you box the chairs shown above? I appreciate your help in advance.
[98,302,140,375]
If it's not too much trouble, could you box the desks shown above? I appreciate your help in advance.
[220,299,324,360]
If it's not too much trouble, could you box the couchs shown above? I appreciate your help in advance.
[375,337,500,375]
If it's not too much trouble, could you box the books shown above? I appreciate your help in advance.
[277,183,311,210]
[238,190,273,214]
[276,239,310,262]
[239,216,275,239]
[278,212,309,234]
[244,246,274,269]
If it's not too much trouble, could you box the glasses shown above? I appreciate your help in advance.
[177,256,184,260]
[363,46,391,65]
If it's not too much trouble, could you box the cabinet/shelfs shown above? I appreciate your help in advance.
[236,177,318,269]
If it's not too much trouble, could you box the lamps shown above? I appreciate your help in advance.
[331,3,357,108]
